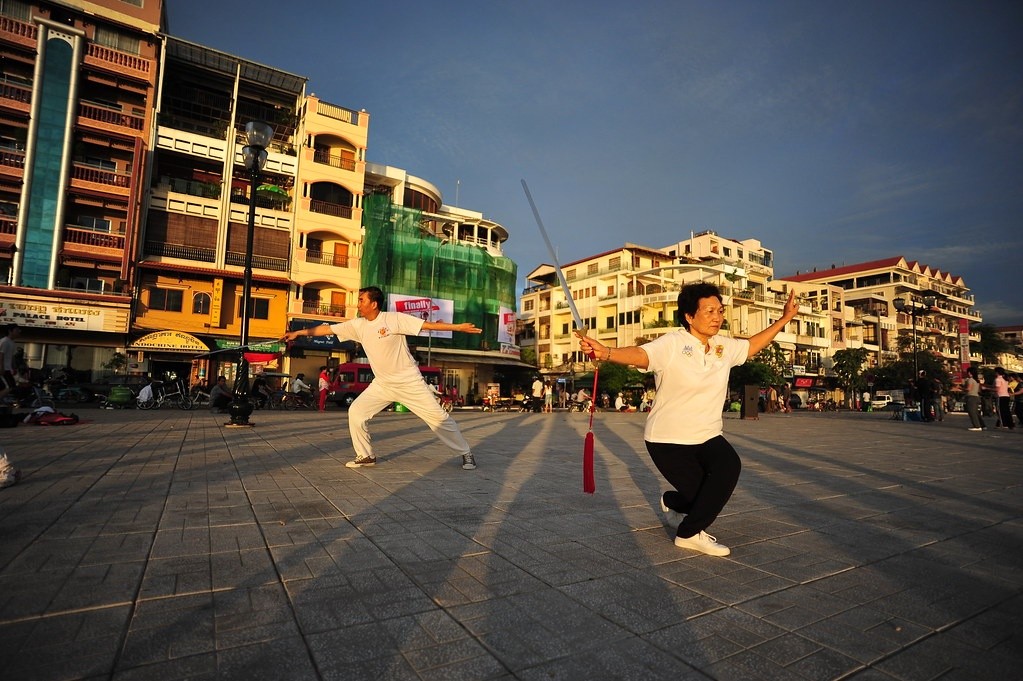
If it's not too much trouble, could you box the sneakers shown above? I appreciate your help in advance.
[346,456,376,467]
[462,454,477,470]
[675,530,730,556]
[660,491,687,528]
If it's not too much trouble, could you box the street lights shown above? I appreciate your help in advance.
[222,93,280,432]
[892,293,935,408]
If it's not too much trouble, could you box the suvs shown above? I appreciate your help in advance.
[788,392,802,410]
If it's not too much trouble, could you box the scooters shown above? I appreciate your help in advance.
[441,393,454,412]
[805,398,839,412]
[481,392,609,413]
[0,368,318,412]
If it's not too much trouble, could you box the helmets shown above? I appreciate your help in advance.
[297,373,304,380]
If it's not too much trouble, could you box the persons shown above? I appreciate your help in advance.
[601,390,610,398]
[640,388,648,411]
[279,286,482,469]
[960,367,987,431]
[294,373,311,408]
[531,377,553,413]
[0,324,19,399]
[575,282,799,555]
[252,370,271,409]
[209,375,232,413]
[615,392,629,412]
[193,375,201,386]
[445,385,464,405]
[770,383,792,413]
[318,366,331,412]
[1007,372,1023,428]
[983,366,1015,429]
[555,388,592,404]
[855,391,861,411]
[915,370,938,422]
[862,390,870,412]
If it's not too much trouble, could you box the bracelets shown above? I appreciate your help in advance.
[606,347,611,360]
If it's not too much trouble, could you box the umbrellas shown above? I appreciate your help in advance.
[256,184,287,203]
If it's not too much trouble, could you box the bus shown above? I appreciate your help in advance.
[325,362,443,408]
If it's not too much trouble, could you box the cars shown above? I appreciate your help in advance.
[871,394,893,410]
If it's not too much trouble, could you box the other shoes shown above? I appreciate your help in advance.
[968,427,986,431]
[259,401,266,405]
[303,402,309,409]
[318,410,328,413]
[0,463,20,489]
